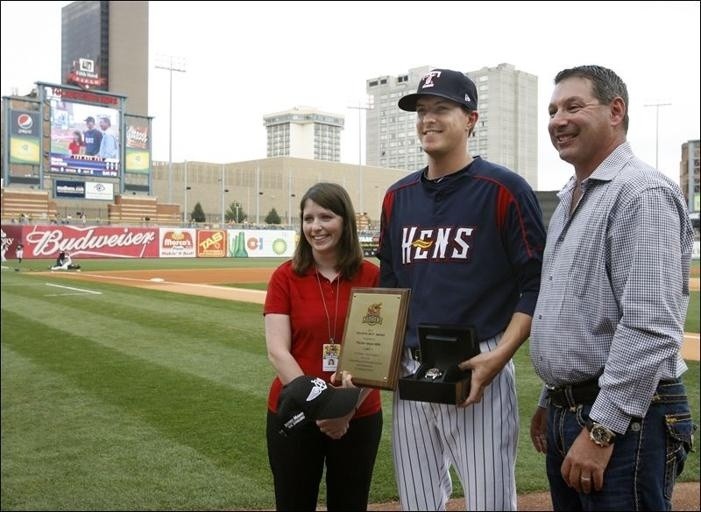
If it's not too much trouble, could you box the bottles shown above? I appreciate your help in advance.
[228,231,248,258]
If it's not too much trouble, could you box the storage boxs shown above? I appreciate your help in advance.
[398,324,475,405]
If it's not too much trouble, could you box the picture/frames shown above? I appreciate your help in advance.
[334,287,412,391]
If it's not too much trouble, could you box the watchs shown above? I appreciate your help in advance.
[585,417,616,448]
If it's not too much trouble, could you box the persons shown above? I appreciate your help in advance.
[82,116,103,156]
[8,210,87,227]
[262,183,384,512]
[69,131,85,154]
[47,248,81,272]
[99,118,117,159]
[15,240,24,263]
[529,65,694,512]
[330,68,546,512]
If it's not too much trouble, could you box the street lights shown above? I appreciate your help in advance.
[288,194,297,225]
[346,104,373,210]
[222,188,230,223]
[256,192,265,224]
[184,184,191,223]
[642,102,674,171]
[155,65,188,203]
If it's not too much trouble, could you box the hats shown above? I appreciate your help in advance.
[277,375,360,435]
[398,68,478,112]
